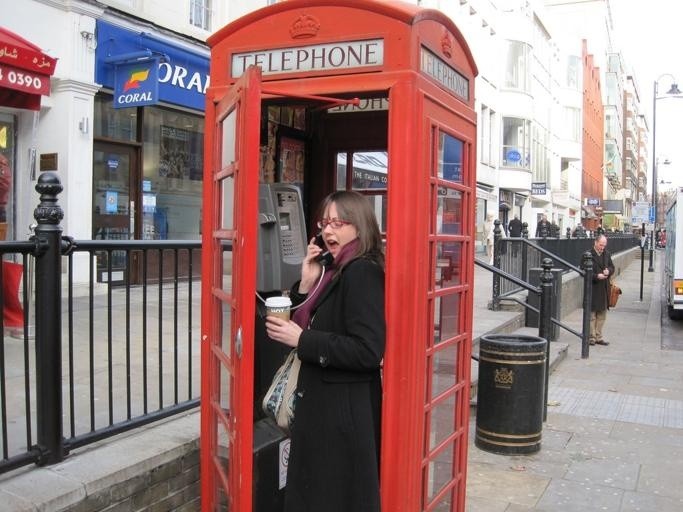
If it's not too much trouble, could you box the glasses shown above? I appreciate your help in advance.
[316,218,354,230]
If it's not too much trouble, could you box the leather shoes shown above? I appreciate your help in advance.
[590,340,595,345]
[595,340,609,346]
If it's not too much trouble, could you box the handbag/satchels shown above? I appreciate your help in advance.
[261,346,301,437]
[609,280,622,308]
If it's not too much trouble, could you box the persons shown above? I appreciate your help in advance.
[483,211,507,267]
[579,233,615,345]
[596,222,605,236]
[0,146,11,227]
[262,188,388,511]
[534,212,552,237]
[507,213,523,260]
[572,221,585,239]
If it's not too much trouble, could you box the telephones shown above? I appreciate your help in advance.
[313,231,334,266]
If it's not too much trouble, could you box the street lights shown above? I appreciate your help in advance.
[648,79,682,273]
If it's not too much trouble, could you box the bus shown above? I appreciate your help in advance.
[663,185,683,321]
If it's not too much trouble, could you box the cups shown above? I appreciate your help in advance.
[263,296,292,322]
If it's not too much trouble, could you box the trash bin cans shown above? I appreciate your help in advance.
[474,334,548,455]
